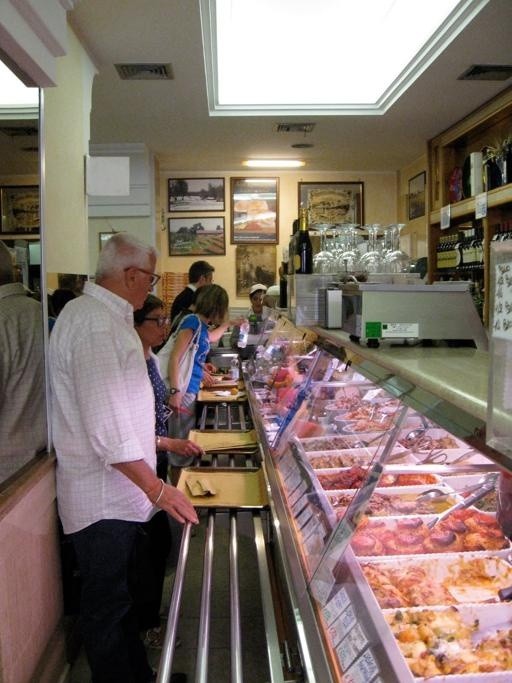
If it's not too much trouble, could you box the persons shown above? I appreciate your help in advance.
[48,232,200,683]
[242,283,271,321]
[133,260,246,482]
[0,240,86,485]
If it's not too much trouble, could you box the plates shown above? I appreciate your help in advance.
[446,166,463,202]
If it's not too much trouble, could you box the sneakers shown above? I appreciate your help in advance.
[144,625,182,649]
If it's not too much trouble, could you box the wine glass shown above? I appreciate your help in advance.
[311,222,410,272]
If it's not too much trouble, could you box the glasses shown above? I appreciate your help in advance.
[124,266,160,287]
[145,316,170,328]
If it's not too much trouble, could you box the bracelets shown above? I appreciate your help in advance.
[152,479,165,506]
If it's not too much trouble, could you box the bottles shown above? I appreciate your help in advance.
[288,207,311,273]
[236,318,250,348]
[249,313,257,334]
[491,219,511,241]
[230,354,240,380]
[474,281,481,299]
[435,226,483,281]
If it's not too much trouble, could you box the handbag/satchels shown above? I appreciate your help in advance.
[157,332,200,399]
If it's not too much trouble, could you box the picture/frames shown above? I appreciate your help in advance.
[297,181,367,229]
[0,185,40,236]
[408,170,426,222]
[168,177,226,212]
[166,216,227,257]
[228,174,281,246]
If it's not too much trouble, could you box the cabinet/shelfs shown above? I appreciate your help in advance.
[241,325,512,683]
[426,80,512,326]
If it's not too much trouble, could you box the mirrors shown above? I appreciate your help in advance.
[0,51,48,493]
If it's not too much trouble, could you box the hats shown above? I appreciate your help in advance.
[249,283,267,294]
